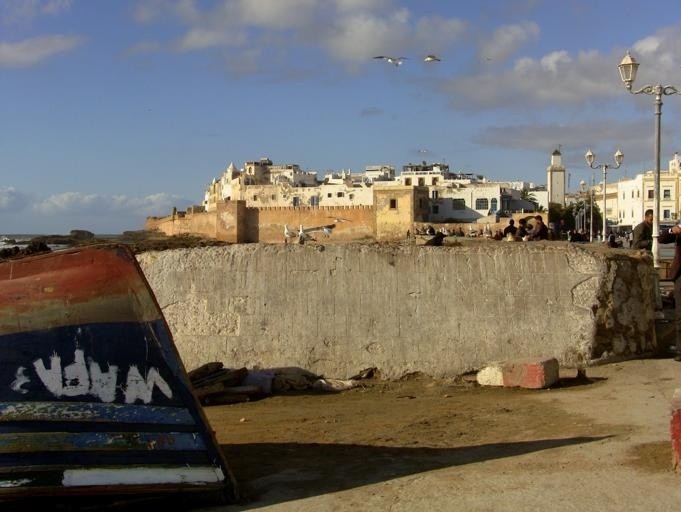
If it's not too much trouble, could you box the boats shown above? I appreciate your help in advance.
[0,239,241,512]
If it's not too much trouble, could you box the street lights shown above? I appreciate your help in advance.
[615,48,681,272]
[579,174,604,244]
[571,188,596,242]
[585,147,625,241]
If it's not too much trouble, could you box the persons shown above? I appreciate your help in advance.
[525,215,550,241]
[657,223,680,354]
[504,219,517,237]
[515,219,530,237]
[633,210,653,251]
[428,225,436,235]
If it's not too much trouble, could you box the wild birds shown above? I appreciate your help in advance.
[523,235,528,242]
[415,224,504,240]
[374,56,410,66]
[322,226,332,238]
[424,55,440,64]
[299,225,312,245]
[284,225,296,241]
[507,232,514,242]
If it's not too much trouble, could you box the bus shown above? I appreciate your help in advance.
[606,224,633,238]
[660,223,676,235]
